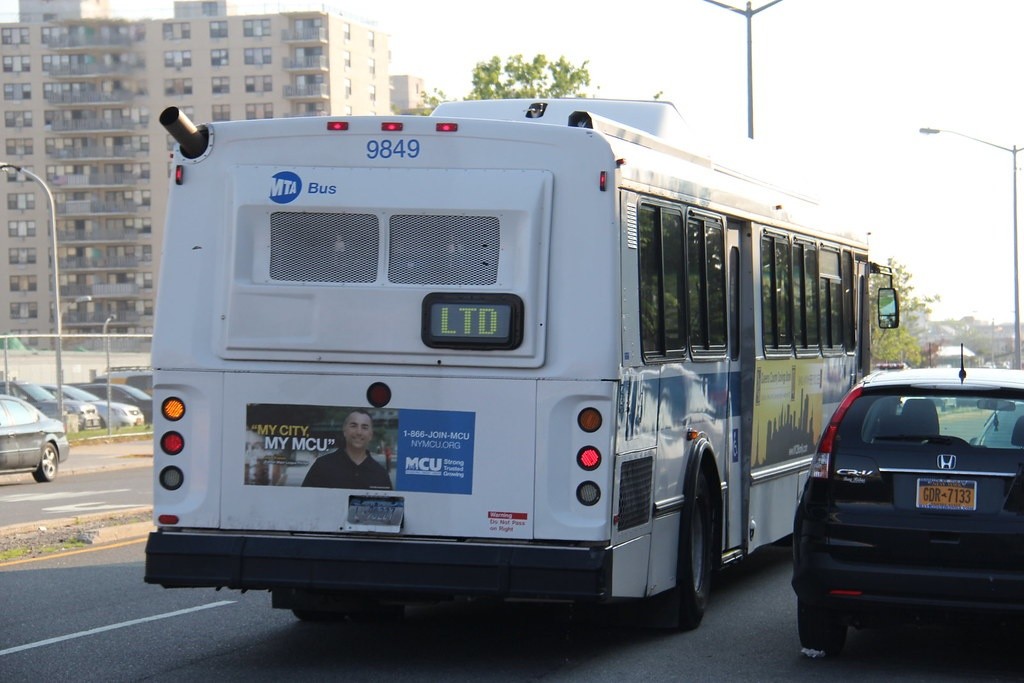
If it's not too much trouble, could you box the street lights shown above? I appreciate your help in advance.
[60,295,93,334]
[1,161,66,426]
[103,314,116,335]
[919,126,1022,370]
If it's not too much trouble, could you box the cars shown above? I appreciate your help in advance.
[39,385,147,428]
[92,370,154,398]
[0,394,69,483]
[65,382,153,426]
[1,380,103,433]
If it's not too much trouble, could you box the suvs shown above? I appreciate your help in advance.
[790,368,1023,659]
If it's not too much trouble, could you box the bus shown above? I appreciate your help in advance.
[139,97,899,630]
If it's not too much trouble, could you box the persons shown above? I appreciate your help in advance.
[385,445,392,475]
[301,411,393,492]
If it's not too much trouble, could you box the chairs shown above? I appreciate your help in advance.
[875,400,939,439]
[1011,416,1024,447]
